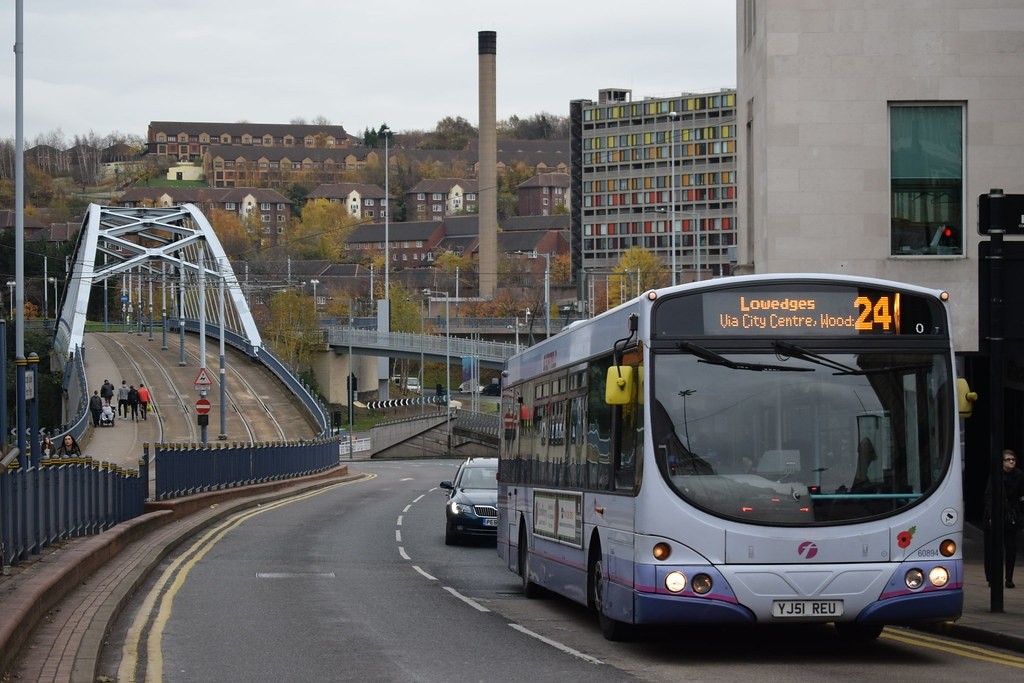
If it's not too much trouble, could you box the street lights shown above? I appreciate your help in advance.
[512,250,549,340]
[7,280,16,321]
[525,307,531,324]
[48,277,57,319]
[310,279,320,314]
[422,288,451,456]
[326,297,352,459]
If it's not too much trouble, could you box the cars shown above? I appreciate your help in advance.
[459,380,501,397]
[390,374,421,393]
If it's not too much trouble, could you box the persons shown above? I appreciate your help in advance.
[436,314,446,329]
[463,314,512,326]
[781,438,816,483]
[117,380,131,421]
[40,435,57,458]
[709,407,754,467]
[137,383,150,420]
[464,470,490,489]
[42,316,51,330]
[858,438,883,483]
[504,397,529,454]
[141,313,149,332]
[55,434,82,458]
[102,400,117,423]
[126,313,130,325]
[128,385,142,423]
[982,449,1024,589]
[659,407,724,475]
[89,390,102,427]
[100,379,114,405]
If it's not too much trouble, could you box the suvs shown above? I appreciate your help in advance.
[441,456,499,546]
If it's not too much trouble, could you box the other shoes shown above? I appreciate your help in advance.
[136,418,139,422]
[1005,580,1015,589]
[118,416,122,419]
[94,425,97,427]
[124,418,127,421]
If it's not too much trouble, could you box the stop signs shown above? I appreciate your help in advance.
[505,413,517,428]
[195,399,211,414]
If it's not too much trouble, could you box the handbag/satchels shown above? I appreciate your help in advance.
[146,404,152,411]
[127,405,130,413]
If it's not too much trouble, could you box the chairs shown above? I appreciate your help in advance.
[470,470,483,482]
[782,438,815,484]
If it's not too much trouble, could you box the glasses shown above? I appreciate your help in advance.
[1003,457,1015,463]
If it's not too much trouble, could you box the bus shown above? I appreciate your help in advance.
[497,273,978,642]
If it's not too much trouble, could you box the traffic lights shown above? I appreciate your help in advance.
[939,223,962,247]
